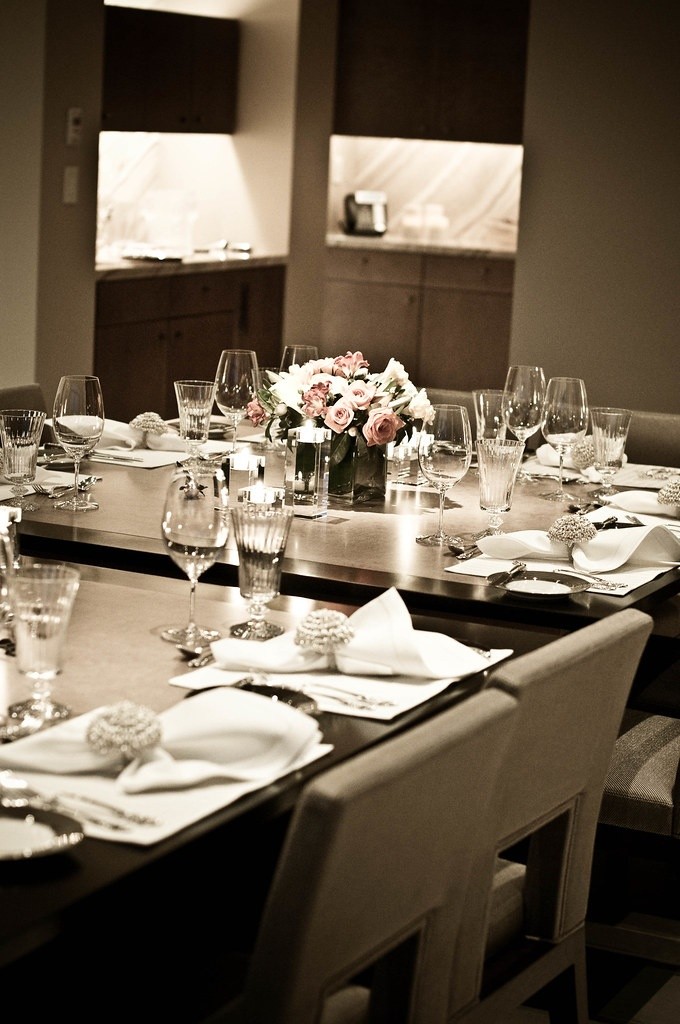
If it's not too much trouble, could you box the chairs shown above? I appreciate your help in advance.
[0,384,679,1024]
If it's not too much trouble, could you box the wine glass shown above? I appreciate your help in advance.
[180,346,634,560]
[0,374,105,737]
[160,467,292,647]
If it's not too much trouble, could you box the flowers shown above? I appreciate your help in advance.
[246,348,437,495]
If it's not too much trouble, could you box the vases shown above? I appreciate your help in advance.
[328,428,389,504]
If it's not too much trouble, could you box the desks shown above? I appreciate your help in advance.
[0,556,560,1024]
[0,440,680,642]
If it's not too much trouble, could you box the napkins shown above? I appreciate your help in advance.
[1,685,324,794]
[536,443,628,483]
[475,524,680,573]
[598,489,680,520]
[386,431,434,460]
[208,585,488,678]
[44,415,226,453]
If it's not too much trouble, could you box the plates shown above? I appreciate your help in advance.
[30,445,92,469]
[486,569,592,600]
[185,681,318,722]
[0,804,85,867]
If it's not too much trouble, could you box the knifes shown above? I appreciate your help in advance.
[484,560,528,593]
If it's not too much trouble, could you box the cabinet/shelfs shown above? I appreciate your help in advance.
[325,242,517,391]
[93,262,291,424]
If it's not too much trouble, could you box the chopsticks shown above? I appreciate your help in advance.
[588,520,645,534]
